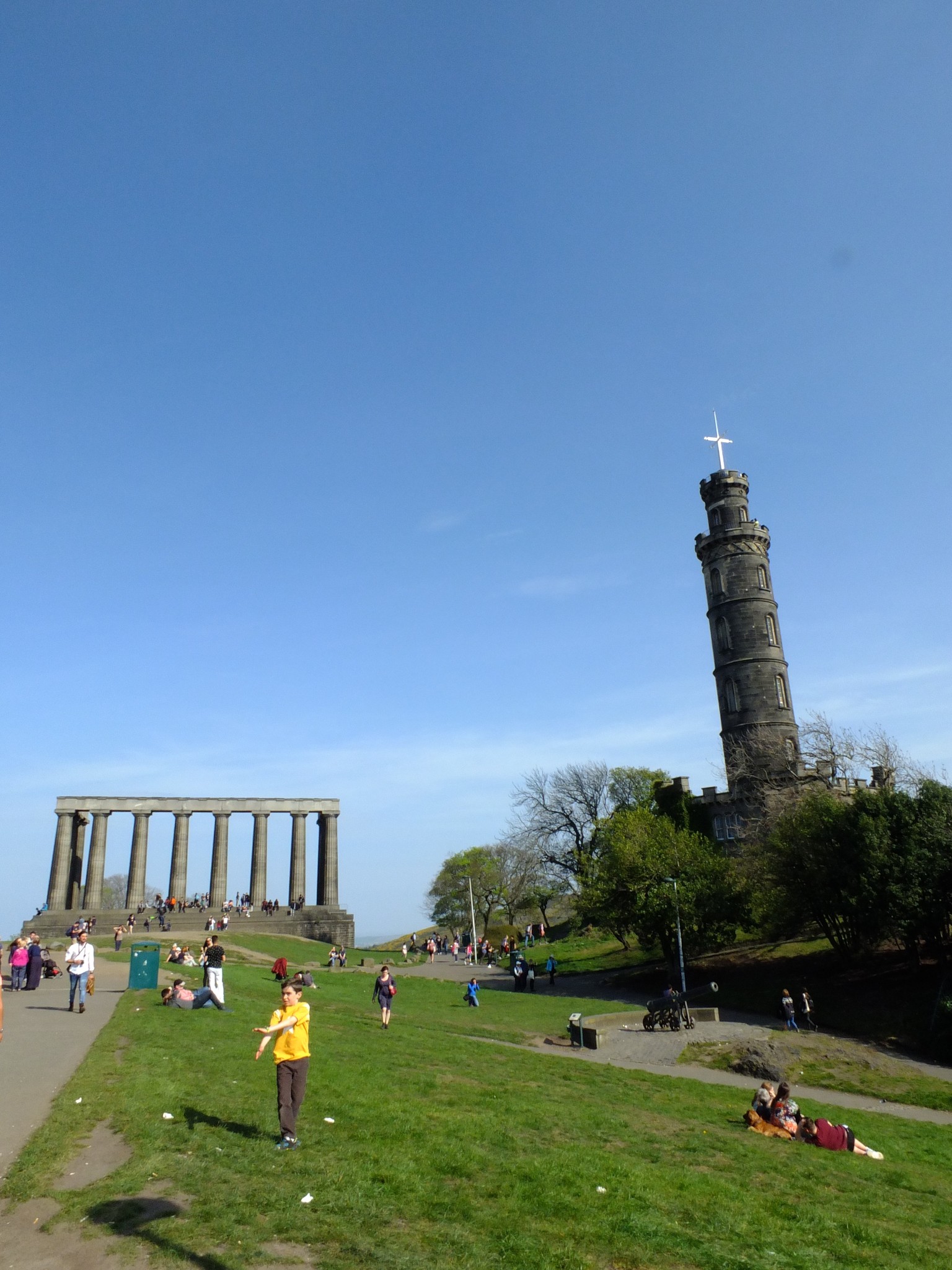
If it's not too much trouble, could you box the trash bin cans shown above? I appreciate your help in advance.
[510,950,526,975]
[461,932,470,947]
[128,941,161,989]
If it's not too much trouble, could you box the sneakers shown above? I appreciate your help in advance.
[274,1137,300,1152]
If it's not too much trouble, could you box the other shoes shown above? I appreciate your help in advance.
[454,952,510,969]
[381,1023,385,1029]
[469,1004,474,1007]
[221,1008,233,1012]
[385,1024,388,1029]
[11,986,36,991]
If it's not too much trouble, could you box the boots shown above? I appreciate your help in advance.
[80,1003,85,1013]
[68,1002,73,1011]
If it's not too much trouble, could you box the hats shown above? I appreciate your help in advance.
[550,955,554,958]
[176,947,182,951]
[516,961,522,965]
[518,955,523,959]
[174,979,185,986]
[161,986,172,998]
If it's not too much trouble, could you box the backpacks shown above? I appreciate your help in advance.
[66,927,72,937]
[525,926,531,932]
[465,948,467,953]
[411,935,413,940]
[209,919,212,925]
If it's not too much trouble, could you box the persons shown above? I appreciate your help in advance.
[756,1088,777,1123]
[798,987,818,1032]
[770,1082,804,1137]
[781,988,801,1034]
[751,1081,778,1118]
[6,890,558,1013]
[251,977,312,1150]
[372,966,396,1030]
[799,1116,885,1160]
[0,975,6,1048]
[661,984,674,997]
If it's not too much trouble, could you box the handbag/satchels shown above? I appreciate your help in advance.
[463,994,470,1001]
[67,964,71,973]
[505,945,509,954]
[388,975,397,995]
[433,946,436,954]
[198,954,205,968]
[86,978,95,996]
[528,967,534,980]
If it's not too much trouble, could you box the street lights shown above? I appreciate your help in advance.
[462,874,478,964]
[663,877,690,1007]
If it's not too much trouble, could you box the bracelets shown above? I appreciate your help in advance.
[0,1028,4,1033]
